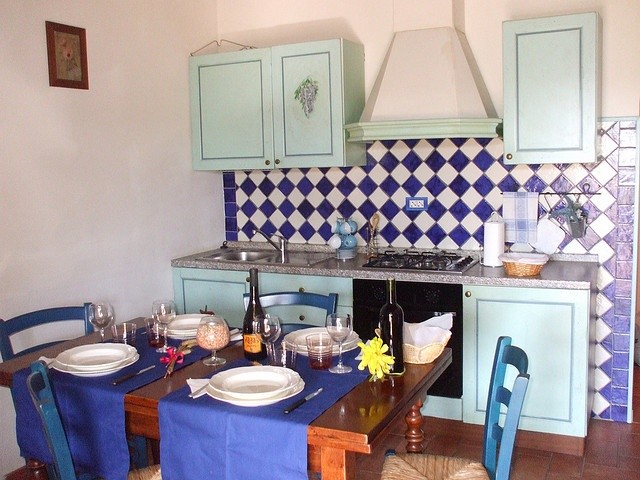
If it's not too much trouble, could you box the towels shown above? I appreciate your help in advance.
[502,191,539,244]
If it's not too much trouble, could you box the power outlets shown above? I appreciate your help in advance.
[409,199,425,208]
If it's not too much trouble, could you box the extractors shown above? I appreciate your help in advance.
[345,0,502,142]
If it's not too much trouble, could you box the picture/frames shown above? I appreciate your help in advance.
[45,21,89,90]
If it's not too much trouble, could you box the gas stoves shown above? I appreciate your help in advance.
[362,250,483,275]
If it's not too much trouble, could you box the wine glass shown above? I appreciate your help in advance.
[253,313,282,365]
[196,315,230,366]
[152,298,177,353]
[88,301,114,343]
[326,313,356,374]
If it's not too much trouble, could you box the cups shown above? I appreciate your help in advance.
[306,333,333,371]
[111,322,137,348]
[274,340,297,369]
[144,317,165,348]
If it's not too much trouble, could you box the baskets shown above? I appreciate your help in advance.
[499,253,549,276]
[375,329,453,364]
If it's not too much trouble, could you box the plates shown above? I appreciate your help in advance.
[165,333,198,340]
[280,338,363,359]
[203,379,306,408]
[50,353,141,378]
[209,366,302,399]
[55,343,138,371]
[164,313,211,333]
[284,326,359,351]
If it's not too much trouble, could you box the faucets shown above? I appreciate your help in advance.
[252,230,288,251]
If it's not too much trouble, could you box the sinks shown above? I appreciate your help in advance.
[265,252,326,266]
[203,250,274,262]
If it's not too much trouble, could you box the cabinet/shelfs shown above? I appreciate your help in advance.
[188,35,366,172]
[462,286,597,457]
[503,11,602,167]
[258,272,353,330]
[172,268,251,333]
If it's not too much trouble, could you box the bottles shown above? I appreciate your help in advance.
[380,275,405,375]
[243,268,268,361]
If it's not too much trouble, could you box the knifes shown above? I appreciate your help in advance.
[284,387,324,415]
[112,364,157,385]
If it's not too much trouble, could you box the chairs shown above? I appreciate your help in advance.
[25,358,162,479]
[0,302,148,469]
[242,291,338,344]
[381,336,531,480]
